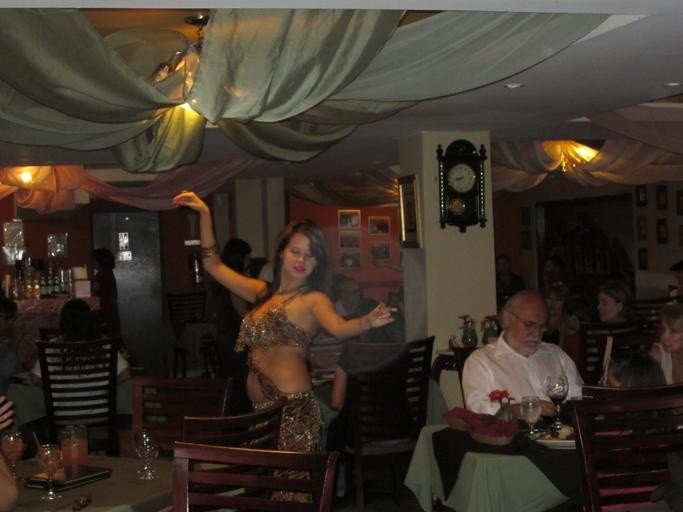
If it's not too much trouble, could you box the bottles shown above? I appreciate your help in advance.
[10,263,74,302]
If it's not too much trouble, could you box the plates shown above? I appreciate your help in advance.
[23,468,110,491]
[533,432,583,450]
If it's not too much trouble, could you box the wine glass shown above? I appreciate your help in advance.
[139,443,161,480]
[519,396,541,436]
[135,429,154,474]
[63,423,87,477]
[0,432,25,481]
[545,375,569,428]
[34,444,63,501]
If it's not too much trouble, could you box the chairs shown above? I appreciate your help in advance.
[0,291,683,512]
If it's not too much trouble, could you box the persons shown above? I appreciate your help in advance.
[0,296,25,391]
[461,291,586,511]
[30,299,131,455]
[91,248,121,336]
[497,254,682,386]
[0,396,19,512]
[172,191,398,502]
[206,239,252,449]
[308,273,404,343]
[592,354,682,504]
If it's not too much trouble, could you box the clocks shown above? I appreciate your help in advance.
[437,139,487,233]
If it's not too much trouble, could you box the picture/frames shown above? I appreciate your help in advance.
[337,172,423,270]
[635,183,683,270]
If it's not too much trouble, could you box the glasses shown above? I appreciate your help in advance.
[511,313,548,333]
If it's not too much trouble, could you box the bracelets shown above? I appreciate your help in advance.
[198,244,218,258]
